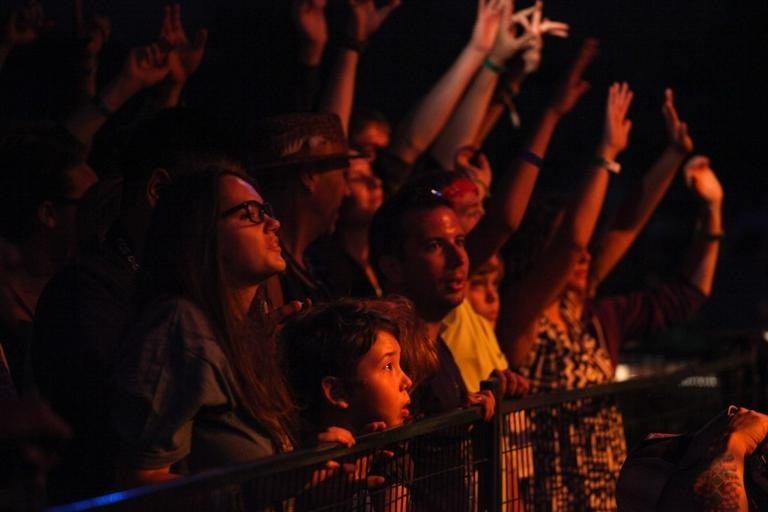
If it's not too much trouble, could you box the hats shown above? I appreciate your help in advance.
[262,112,370,172]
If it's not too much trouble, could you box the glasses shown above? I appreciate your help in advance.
[216,202,275,224]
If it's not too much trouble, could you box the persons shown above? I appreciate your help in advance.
[0,0,768,512]
